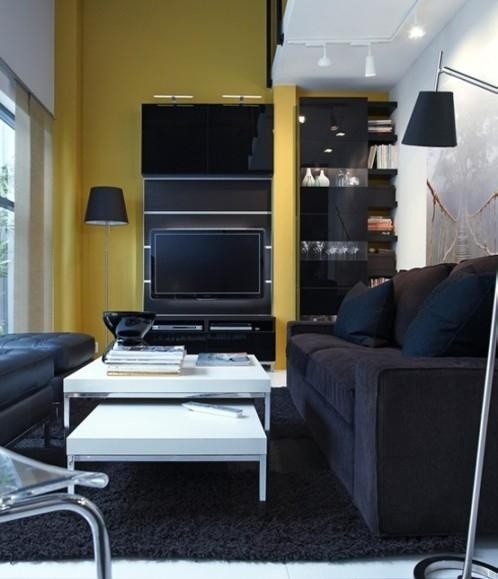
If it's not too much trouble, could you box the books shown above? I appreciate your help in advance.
[195,352,251,367]
[105,343,186,377]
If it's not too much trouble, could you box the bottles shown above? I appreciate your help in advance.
[301,167,331,186]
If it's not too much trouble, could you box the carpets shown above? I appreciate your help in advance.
[0,386,466,559]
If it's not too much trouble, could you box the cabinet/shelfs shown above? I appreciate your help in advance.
[299,96,397,326]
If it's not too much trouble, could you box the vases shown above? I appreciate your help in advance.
[302,168,315,187]
[315,168,330,187]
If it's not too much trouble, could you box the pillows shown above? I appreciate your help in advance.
[331,256,497,357]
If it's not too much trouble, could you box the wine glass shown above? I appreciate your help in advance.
[299,240,359,262]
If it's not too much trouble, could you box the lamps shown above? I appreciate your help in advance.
[401,50,498,147]
[83,186,129,354]
[285,0,426,77]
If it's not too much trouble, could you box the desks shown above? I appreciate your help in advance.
[144,315,276,363]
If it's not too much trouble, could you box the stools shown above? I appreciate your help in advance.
[0,446,112,579]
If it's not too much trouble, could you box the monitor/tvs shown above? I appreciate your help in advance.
[151,230,263,298]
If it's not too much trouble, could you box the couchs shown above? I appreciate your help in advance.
[0,332,95,415]
[286,256,498,539]
[0,348,54,449]
